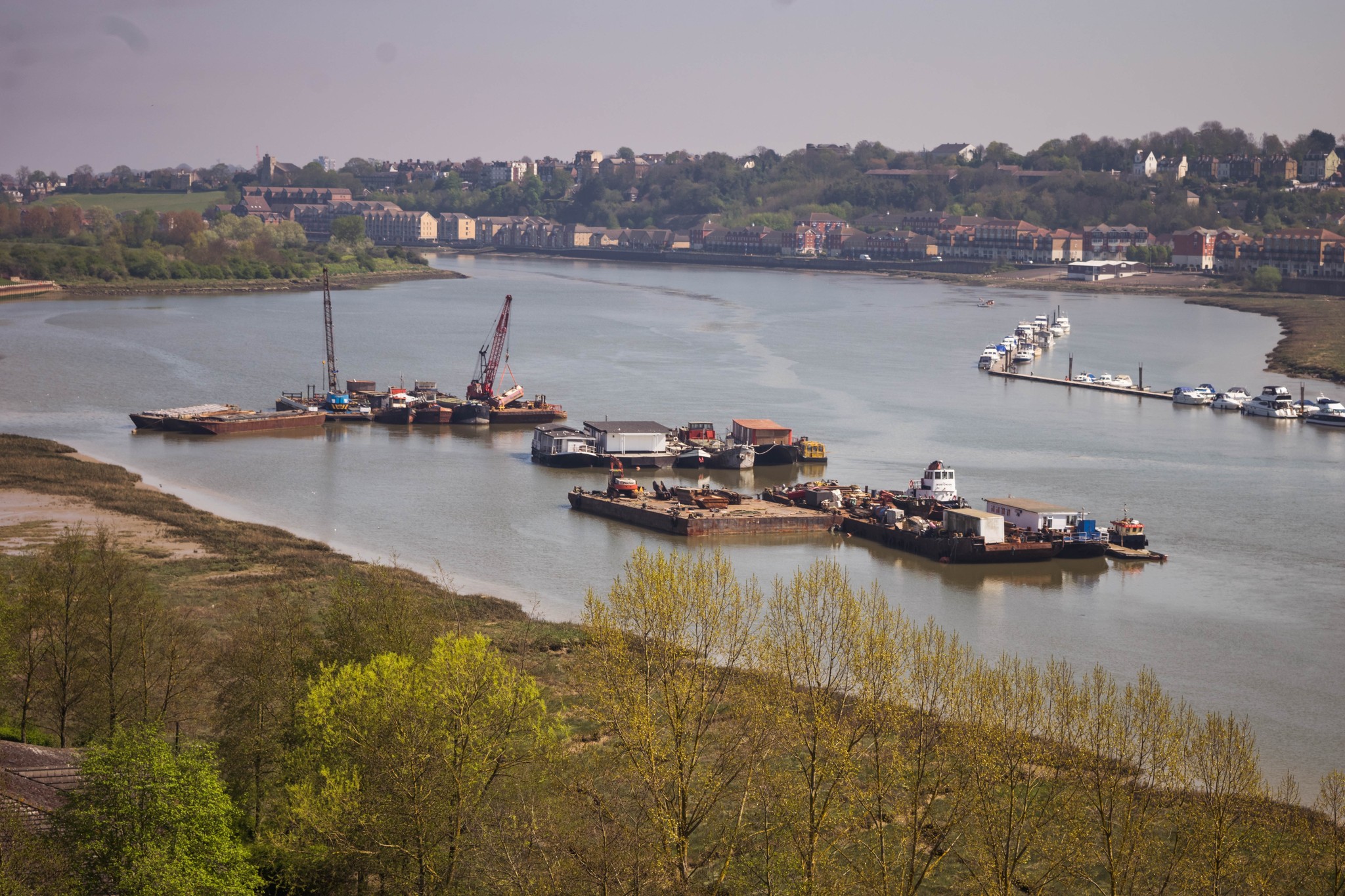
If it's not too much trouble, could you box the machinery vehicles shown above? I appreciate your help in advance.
[607,453,638,499]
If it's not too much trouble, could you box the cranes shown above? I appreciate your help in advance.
[465,294,513,399]
[322,267,338,393]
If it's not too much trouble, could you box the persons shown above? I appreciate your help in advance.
[315,394,317,398]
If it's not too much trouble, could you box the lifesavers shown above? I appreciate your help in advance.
[673,437,678,441]
[909,480,913,487]
[1301,408,1304,413]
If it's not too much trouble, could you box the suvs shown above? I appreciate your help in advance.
[1023,259,1033,264]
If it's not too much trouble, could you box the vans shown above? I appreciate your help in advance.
[860,254,871,261]
[931,256,942,262]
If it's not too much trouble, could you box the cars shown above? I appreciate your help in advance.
[1203,269,1214,274]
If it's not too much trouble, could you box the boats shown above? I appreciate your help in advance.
[553,405,568,419]
[1071,371,1133,388]
[410,401,453,424]
[1300,393,1345,427]
[694,496,730,510]
[531,422,597,467]
[710,444,755,469]
[325,391,349,411]
[371,386,410,424]
[977,313,1071,368]
[798,438,828,463]
[904,459,972,509]
[452,395,491,424]
[1172,384,1298,418]
[675,447,711,468]
[128,403,326,435]
[1094,502,1149,550]
